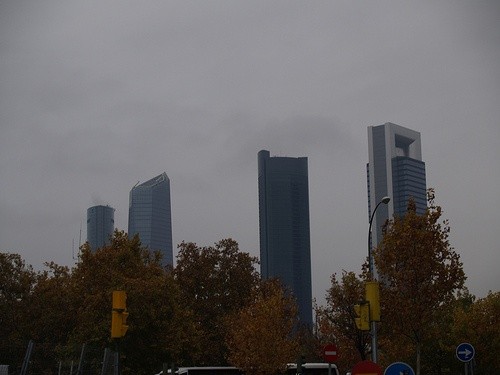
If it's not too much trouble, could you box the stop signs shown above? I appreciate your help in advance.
[322,344,339,363]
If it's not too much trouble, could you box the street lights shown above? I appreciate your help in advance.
[364,196,392,365]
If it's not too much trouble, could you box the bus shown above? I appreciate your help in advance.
[154,362,339,375]
